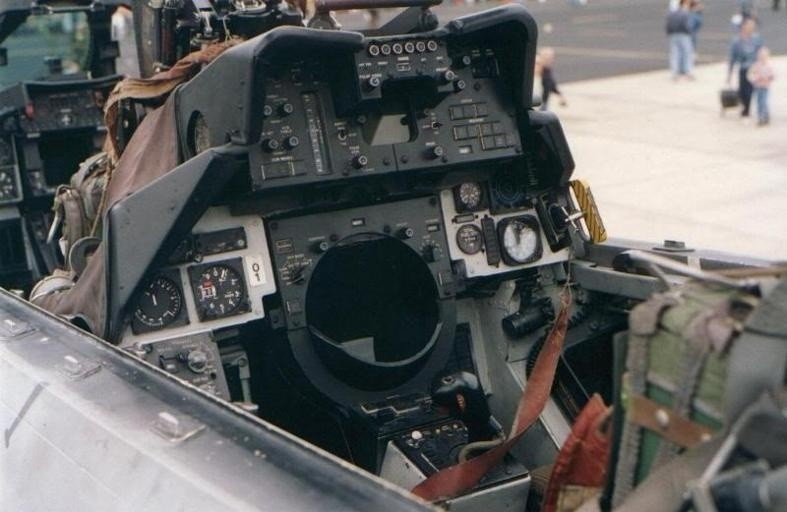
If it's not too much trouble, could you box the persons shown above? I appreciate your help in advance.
[665,0,777,128]
[530,53,544,114]
[540,46,567,111]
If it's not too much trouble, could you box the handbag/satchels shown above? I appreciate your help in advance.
[721,90,736,107]
